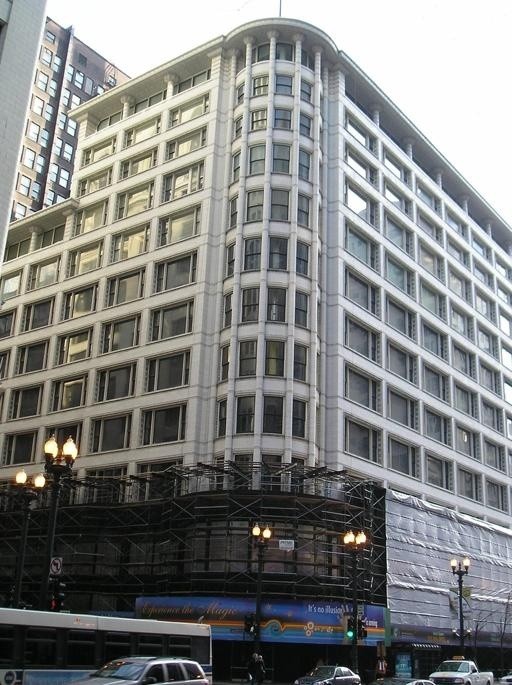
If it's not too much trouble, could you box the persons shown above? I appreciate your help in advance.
[256,655,264,671]
[248,653,265,685]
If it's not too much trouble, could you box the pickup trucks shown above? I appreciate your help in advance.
[427,657,494,685]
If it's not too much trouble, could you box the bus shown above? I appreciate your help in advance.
[1,608,213,685]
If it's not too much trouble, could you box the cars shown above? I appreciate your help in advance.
[294,665,362,685]
[371,678,437,685]
[498,674,512,685]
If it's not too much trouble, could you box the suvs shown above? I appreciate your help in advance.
[68,657,209,685]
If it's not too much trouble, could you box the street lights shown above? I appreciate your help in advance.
[43,431,78,588]
[12,468,47,609]
[342,528,368,676]
[252,520,272,654]
[449,557,470,655]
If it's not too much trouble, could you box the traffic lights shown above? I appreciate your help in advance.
[54,576,67,600]
[249,625,257,634]
[346,618,356,640]
[49,598,61,612]
